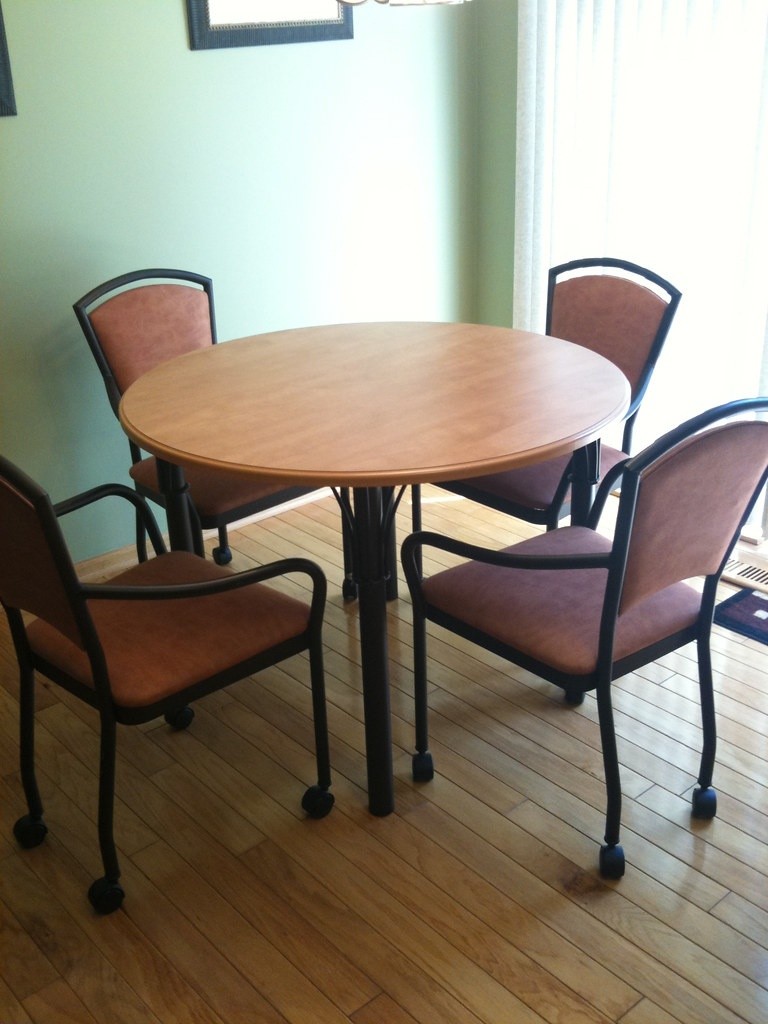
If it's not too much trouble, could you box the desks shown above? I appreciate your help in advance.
[117,320,634,820]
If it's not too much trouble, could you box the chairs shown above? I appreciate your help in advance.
[0,454,335,917]
[72,269,355,608]
[385,258,683,603]
[402,395,768,881]
